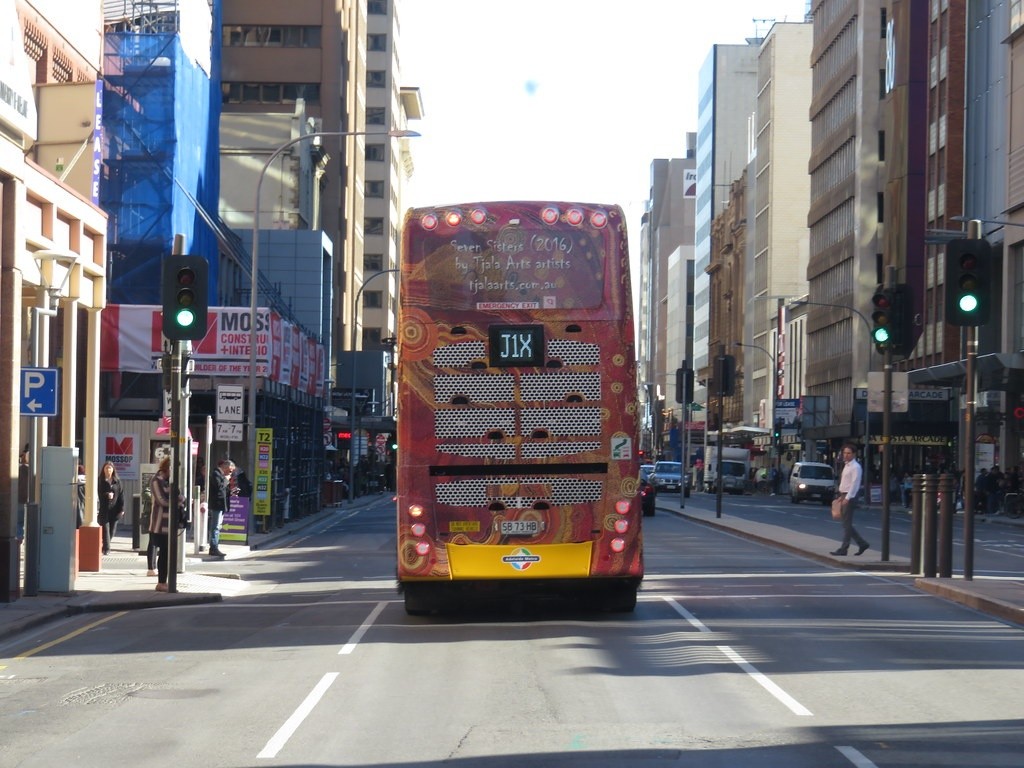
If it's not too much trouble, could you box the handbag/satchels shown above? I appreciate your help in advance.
[831,497,842,522]
[179,507,190,528]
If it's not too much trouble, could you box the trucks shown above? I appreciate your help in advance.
[695,446,751,495]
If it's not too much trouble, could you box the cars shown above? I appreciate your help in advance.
[640,465,655,478]
[649,460,693,498]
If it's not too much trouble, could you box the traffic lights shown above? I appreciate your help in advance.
[871,283,912,356]
[943,237,992,326]
[774,424,780,438]
[162,254,209,341]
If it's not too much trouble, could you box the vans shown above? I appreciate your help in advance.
[789,462,839,506]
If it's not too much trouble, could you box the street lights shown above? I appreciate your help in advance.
[246,129,422,531]
[790,298,892,563]
[734,342,776,496]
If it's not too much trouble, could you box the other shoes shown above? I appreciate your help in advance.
[147,569,158,576]
[156,582,178,593]
[209,545,226,557]
[103,548,109,555]
[830,548,848,556]
[854,544,870,556]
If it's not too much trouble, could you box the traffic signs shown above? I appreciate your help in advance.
[19,367,58,416]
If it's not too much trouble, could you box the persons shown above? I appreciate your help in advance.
[326,453,392,498]
[872,464,1024,517]
[99,461,125,555]
[830,444,870,555]
[201,460,240,557]
[75,458,85,528]
[142,455,187,594]
[19,441,31,546]
[693,458,705,492]
[750,463,790,497]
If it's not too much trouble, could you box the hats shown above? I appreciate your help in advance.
[78,465,84,474]
[23,443,28,453]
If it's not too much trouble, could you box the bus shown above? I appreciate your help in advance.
[395,199,644,616]
